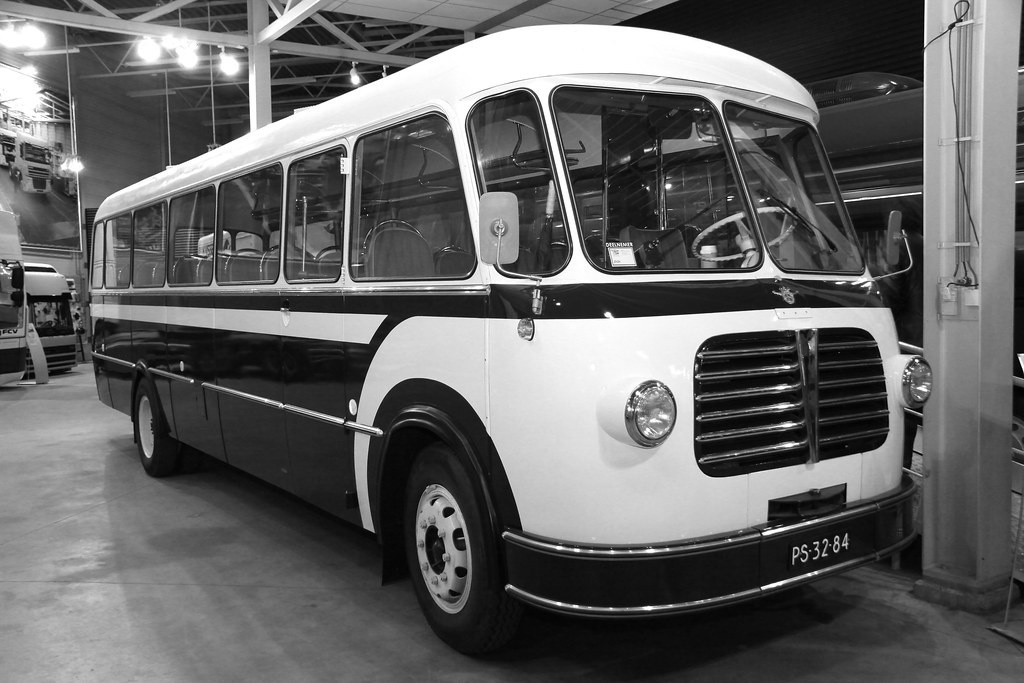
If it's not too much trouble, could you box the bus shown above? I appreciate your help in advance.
[85,23,935,661]
[330,69,1024,584]
[21,259,77,378]
[0,205,30,388]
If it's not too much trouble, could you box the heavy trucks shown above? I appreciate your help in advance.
[0,127,53,194]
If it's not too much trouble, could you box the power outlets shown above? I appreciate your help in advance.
[940,277,959,302]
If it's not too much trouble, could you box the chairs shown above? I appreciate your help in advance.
[314,246,342,279]
[434,246,475,276]
[619,225,689,269]
[259,244,318,280]
[173,255,207,283]
[152,256,182,285]
[223,248,264,281]
[363,219,434,277]
[118,264,131,286]
[503,232,620,274]
[135,260,164,286]
[196,252,231,282]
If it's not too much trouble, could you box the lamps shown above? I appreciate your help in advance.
[219,51,237,75]
[350,68,360,86]
